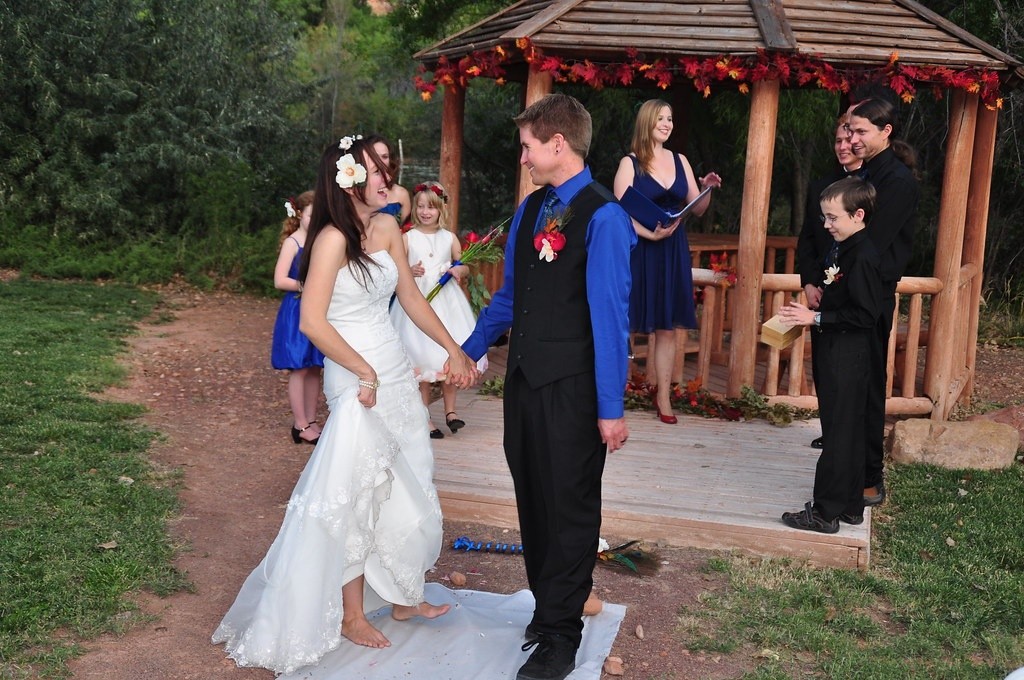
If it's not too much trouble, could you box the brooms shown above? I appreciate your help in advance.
[451,535,660,580]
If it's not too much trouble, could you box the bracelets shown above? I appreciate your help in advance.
[358,380,380,390]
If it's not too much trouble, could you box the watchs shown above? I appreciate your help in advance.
[814,312,821,326]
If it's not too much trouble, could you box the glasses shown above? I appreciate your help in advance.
[819,211,858,223]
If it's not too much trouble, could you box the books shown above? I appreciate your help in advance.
[619,185,711,232]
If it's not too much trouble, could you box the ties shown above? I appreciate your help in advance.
[534,192,559,235]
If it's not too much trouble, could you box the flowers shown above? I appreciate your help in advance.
[425,215,514,321]
[335,134,367,189]
[531,204,576,264]
[822,263,849,286]
[283,197,298,218]
[412,184,448,204]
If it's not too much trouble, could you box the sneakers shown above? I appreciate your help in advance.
[525,608,545,640]
[781,502,840,533]
[516,634,575,679]
[839,506,863,525]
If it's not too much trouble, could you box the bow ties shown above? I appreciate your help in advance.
[841,169,857,178]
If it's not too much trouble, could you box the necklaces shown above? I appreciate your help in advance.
[421,228,438,257]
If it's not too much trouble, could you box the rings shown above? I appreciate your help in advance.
[620,439,626,443]
[455,384,460,387]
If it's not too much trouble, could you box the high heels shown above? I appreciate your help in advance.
[445,411,466,434]
[653,394,677,424]
[428,417,444,439]
[292,425,320,445]
[308,421,322,434]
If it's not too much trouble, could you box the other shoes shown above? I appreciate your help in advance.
[811,437,823,448]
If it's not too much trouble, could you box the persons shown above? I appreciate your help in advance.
[443,100,638,680]
[211,135,477,675]
[796,114,864,449]
[271,190,325,445]
[613,100,721,425]
[849,98,922,506]
[373,181,489,439]
[778,175,884,534]
[366,137,412,312]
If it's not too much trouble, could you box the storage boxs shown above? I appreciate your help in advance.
[759,314,802,350]
